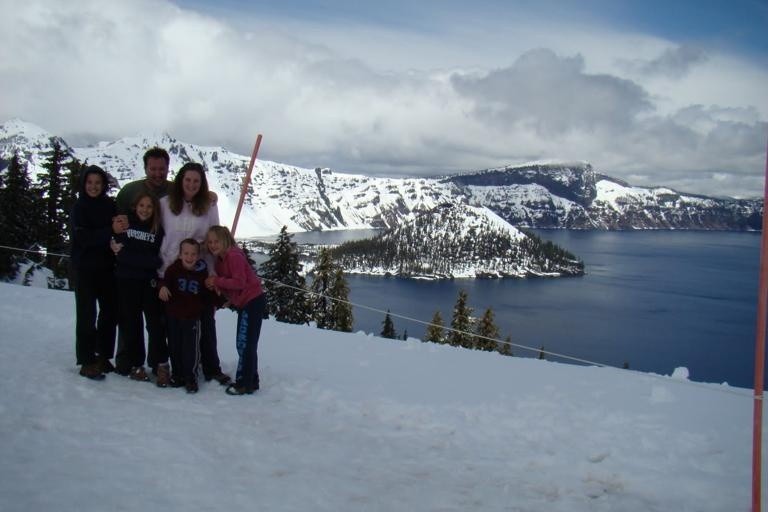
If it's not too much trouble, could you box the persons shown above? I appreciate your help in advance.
[110,191,163,382]
[110,162,230,385]
[113,147,218,375]
[156,238,216,395]
[204,225,266,396]
[68,165,122,380]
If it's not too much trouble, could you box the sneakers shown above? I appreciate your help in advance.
[80,359,259,395]
